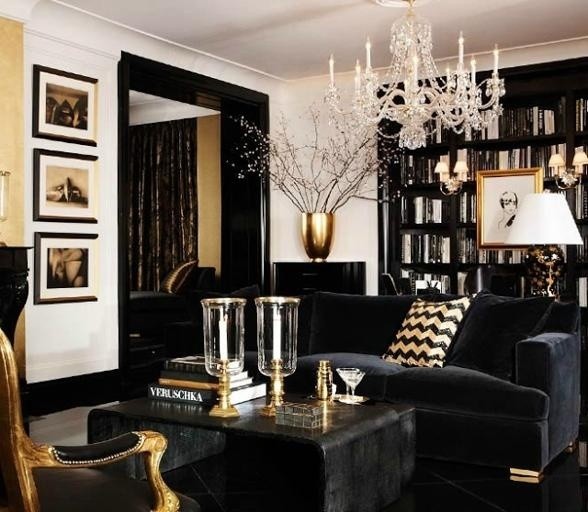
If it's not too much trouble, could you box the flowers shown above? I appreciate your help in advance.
[225,96,417,214]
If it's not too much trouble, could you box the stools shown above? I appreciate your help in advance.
[308,394,417,484]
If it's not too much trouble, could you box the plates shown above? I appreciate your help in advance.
[334,393,370,404]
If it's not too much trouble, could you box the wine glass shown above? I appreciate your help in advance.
[335,367,358,398]
[253,296,301,415]
[342,372,364,400]
[200,296,245,418]
[0,169,11,247]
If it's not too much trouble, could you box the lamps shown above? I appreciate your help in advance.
[323,0,506,150]
[546,151,588,190]
[503,190,584,301]
[434,161,470,195]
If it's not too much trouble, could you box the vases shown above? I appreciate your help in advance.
[301,211,337,263]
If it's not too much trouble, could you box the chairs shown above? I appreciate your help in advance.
[1,326,203,511]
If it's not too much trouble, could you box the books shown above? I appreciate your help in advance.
[147,356,267,406]
[400,98,588,307]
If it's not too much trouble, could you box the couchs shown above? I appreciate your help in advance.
[127,266,218,339]
[162,293,583,481]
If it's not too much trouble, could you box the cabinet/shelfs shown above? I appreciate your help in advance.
[273,260,367,299]
[376,57,587,307]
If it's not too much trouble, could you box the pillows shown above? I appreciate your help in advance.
[159,258,201,295]
[381,294,478,367]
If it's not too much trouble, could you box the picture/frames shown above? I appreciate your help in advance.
[33,146,100,223]
[474,168,544,252]
[31,63,99,148]
[33,231,100,305]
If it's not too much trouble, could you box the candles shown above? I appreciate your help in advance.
[272,311,286,361]
[217,313,230,363]
